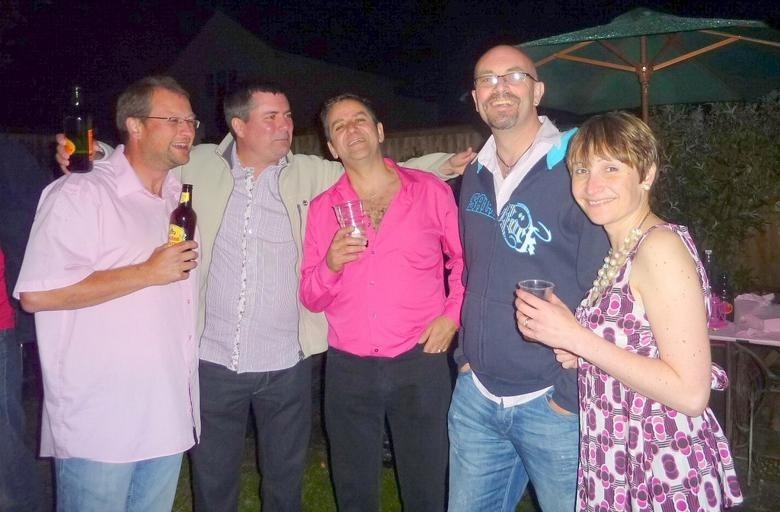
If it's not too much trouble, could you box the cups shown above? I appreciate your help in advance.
[515,279,555,343]
[332,200,371,247]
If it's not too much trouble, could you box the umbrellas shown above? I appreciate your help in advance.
[516,8,780,124]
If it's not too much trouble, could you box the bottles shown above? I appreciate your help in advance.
[167,183,197,274]
[718,274,735,322]
[702,249,716,291]
[66,84,94,172]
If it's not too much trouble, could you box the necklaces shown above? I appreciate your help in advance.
[593,208,654,287]
[496,142,535,177]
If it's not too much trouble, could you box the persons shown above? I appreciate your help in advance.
[448,45,612,510]
[301,91,465,512]
[1,139,47,463]
[0,253,40,512]
[513,111,745,511]
[56,67,477,512]
[10,73,201,511]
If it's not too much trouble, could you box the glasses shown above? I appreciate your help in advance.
[473,70,538,87]
[143,114,202,130]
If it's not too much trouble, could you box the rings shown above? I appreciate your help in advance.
[522,317,533,329]
[436,346,444,356]
